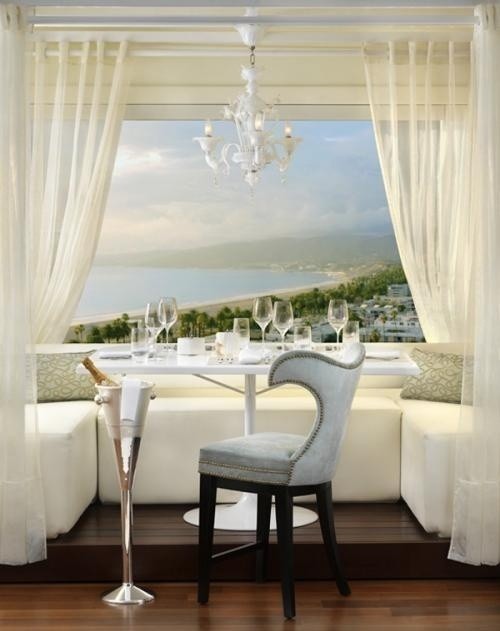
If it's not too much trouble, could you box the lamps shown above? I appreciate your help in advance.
[192,7,304,188]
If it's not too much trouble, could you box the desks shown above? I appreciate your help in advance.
[75,348,420,532]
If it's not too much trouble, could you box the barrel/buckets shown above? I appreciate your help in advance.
[93,377,156,439]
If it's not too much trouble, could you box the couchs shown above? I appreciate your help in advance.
[24,340,475,540]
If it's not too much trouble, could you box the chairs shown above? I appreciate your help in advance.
[197,343,366,620]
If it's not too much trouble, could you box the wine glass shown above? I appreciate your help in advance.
[157,296,178,352]
[327,299,349,356]
[272,300,294,356]
[144,301,166,360]
[252,296,273,358]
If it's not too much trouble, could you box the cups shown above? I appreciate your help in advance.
[343,320,359,352]
[137,316,155,347]
[233,318,250,351]
[293,326,311,351]
[131,328,148,363]
[216,332,238,364]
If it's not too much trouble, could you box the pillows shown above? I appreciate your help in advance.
[399,346,488,407]
[15,348,100,404]
